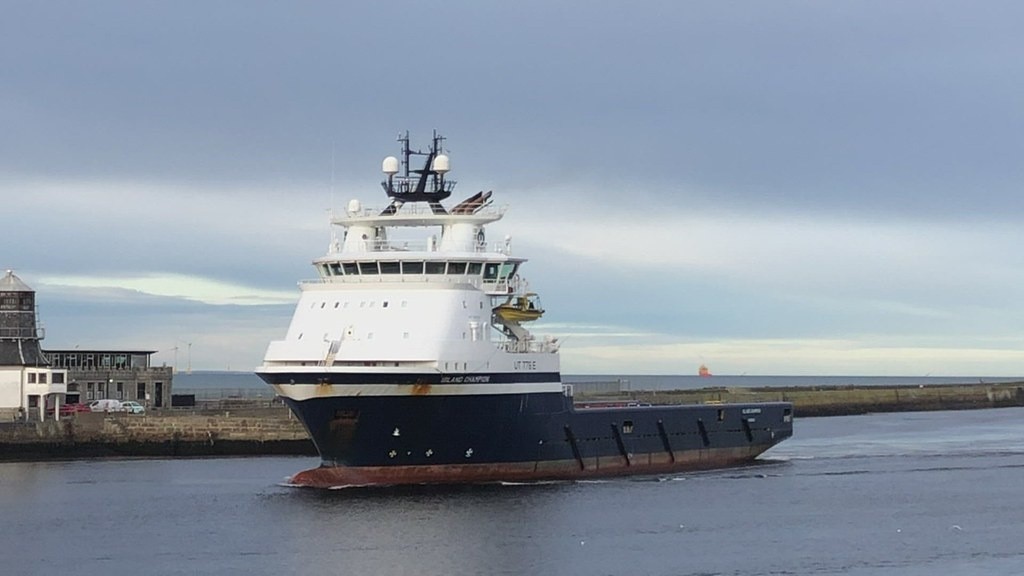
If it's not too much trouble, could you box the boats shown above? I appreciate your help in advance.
[491,291,545,325]
[253,127,796,491]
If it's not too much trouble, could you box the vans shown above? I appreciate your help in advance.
[90,398,122,412]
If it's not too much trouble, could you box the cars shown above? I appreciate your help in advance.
[76,401,93,412]
[47,404,76,416]
[119,401,145,412]
